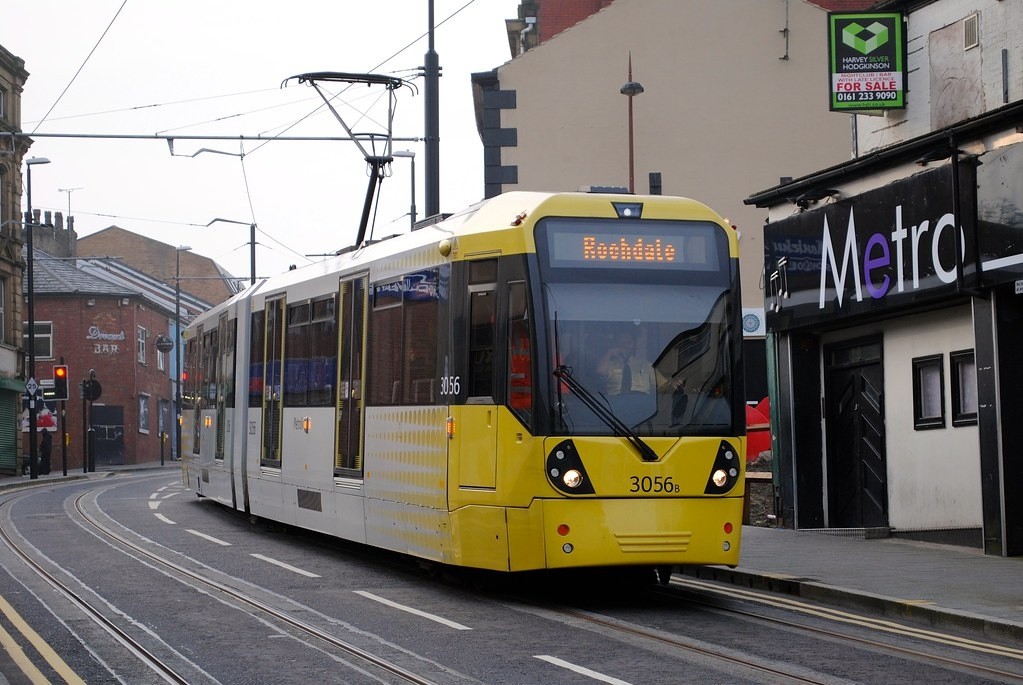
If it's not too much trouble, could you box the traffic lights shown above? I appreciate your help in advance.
[43,365,69,401]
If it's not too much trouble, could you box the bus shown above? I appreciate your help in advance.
[180,72,747,572]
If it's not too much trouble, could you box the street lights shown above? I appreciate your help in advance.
[392,149,416,231]
[175,245,193,461]
[621,51,646,194]
[26,156,51,479]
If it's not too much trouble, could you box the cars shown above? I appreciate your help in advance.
[23,454,43,476]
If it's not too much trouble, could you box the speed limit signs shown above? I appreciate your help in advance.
[25,377,39,396]
